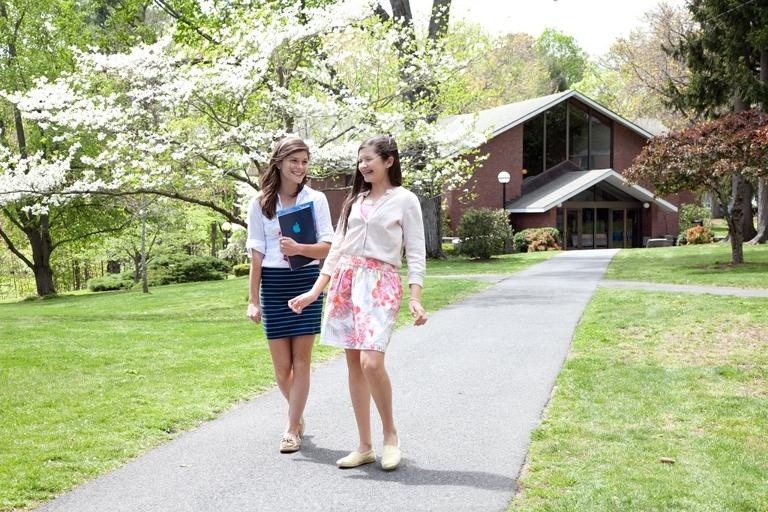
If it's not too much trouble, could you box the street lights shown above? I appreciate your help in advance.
[498,170,515,254]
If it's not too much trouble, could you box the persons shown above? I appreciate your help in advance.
[245,136,335,453]
[288,136,428,470]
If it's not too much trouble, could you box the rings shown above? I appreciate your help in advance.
[422,322,425,324]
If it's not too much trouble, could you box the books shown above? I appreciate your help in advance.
[276,201,321,266]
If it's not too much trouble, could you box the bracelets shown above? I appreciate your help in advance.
[409,297,422,303]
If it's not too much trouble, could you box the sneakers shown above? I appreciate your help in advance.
[380,429,402,470]
[336,450,376,468]
[280,414,305,452]
